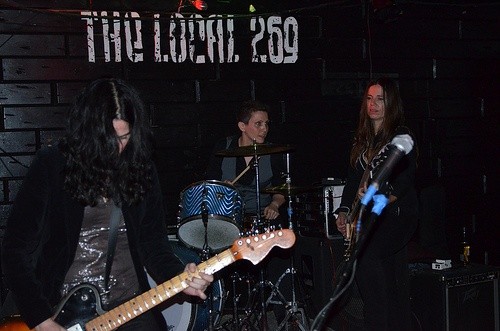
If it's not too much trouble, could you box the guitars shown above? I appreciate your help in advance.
[0,222,297,330]
[342,137,391,264]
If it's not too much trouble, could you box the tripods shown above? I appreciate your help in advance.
[213,153,309,331]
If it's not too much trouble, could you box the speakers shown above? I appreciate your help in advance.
[410,274,497,331]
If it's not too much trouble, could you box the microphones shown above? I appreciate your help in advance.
[361,133,415,207]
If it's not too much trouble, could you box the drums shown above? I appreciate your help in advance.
[142,244,224,331]
[175,179,244,251]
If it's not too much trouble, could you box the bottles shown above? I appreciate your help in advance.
[462,226,470,266]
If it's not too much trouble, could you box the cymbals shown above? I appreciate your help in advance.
[208,143,297,158]
[256,183,323,194]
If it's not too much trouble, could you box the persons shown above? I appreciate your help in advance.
[335,79,420,331]
[205,102,294,323]
[1,78,214,331]
